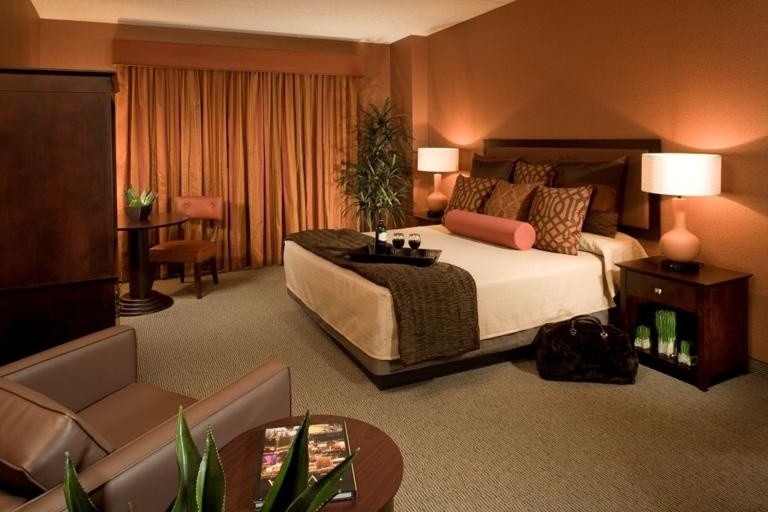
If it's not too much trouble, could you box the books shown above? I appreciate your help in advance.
[254,422,357,510]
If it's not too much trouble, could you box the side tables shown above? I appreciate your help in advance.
[191,414,405,512]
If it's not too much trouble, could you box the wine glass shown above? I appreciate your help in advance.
[392,233,405,257]
[408,233,421,259]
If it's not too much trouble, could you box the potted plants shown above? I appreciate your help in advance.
[122,185,157,223]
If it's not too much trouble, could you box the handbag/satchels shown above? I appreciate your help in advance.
[532,315,638,384]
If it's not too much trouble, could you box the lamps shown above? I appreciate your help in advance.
[640,150,722,272]
[417,147,460,217]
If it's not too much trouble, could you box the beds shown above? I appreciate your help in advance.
[280,137,663,391]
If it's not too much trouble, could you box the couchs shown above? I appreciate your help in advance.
[0,325,293,512]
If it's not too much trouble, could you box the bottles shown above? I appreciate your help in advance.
[375,211,387,255]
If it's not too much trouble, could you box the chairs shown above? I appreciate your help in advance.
[147,196,225,299]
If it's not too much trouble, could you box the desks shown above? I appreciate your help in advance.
[117,212,190,317]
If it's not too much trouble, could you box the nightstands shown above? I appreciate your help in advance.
[406,213,442,227]
[614,256,754,393]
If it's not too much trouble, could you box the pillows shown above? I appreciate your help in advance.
[512,158,553,185]
[444,210,537,251]
[446,173,498,213]
[552,155,631,239]
[477,178,541,220]
[470,151,524,181]
[526,184,594,255]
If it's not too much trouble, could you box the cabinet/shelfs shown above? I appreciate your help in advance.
[1,67,121,362]
